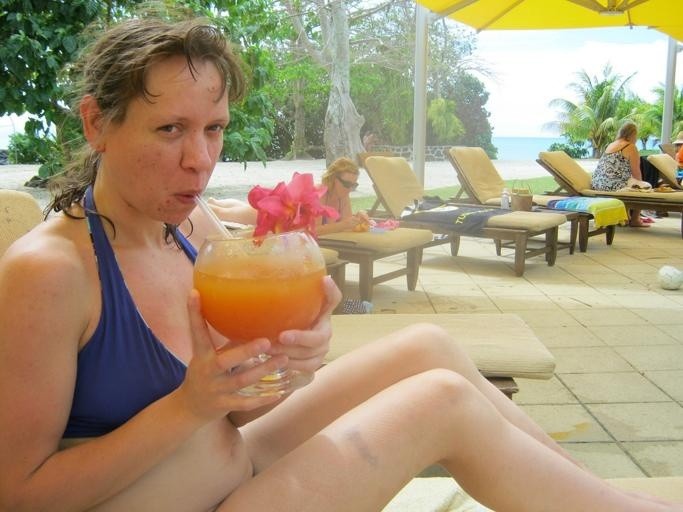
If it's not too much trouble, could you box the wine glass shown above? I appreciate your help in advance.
[192,230,326,398]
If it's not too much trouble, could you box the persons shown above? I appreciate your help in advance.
[0,13,683,511]
[671,130,682,186]
[591,118,657,230]
[309,157,374,236]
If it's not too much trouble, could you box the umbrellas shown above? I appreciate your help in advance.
[409,0,683,203]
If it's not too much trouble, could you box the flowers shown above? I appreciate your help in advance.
[249,169,345,249]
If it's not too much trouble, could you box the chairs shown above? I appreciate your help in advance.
[639,151,682,187]
[657,143,681,160]
[535,148,683,236]
[0,187,682,511]
[358,147,567,278]
[315,304,559,409]
[211,196,434,305]
[442,143,629,255]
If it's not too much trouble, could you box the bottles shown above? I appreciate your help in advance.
[500,188,510,210]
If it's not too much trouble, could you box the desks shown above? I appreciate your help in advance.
[493,207,581,263]
[322,246,350,302]
